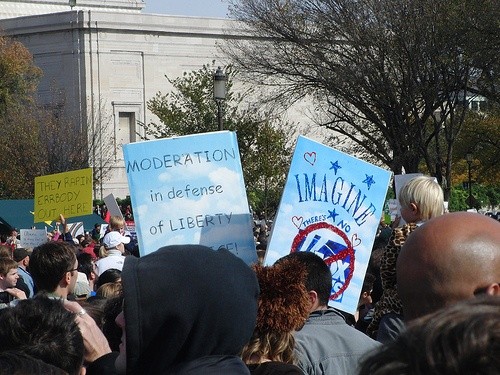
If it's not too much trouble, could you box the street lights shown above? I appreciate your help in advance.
[213,66,226,131]
[466,145,474,209]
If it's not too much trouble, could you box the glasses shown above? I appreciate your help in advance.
[65,264,83,273]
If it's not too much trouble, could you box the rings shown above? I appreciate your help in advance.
[77,309,86,317]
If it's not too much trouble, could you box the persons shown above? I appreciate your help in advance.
[239,261,313,375]
[94,202,134,222]
[0,294,114,375]
[251,207,276,255]
[354,294,500,375]
[82,268,124,345]
[113,245,260,375]
[338,210,408,348]
[366,176,444,343]
[271,251,387,375]
[478,209,498,220]
[394,211,500,325]
[0,214,139,303]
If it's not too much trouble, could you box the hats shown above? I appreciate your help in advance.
[13,248,29,262]
[95,223,101,228]
[103,231,130,249]
